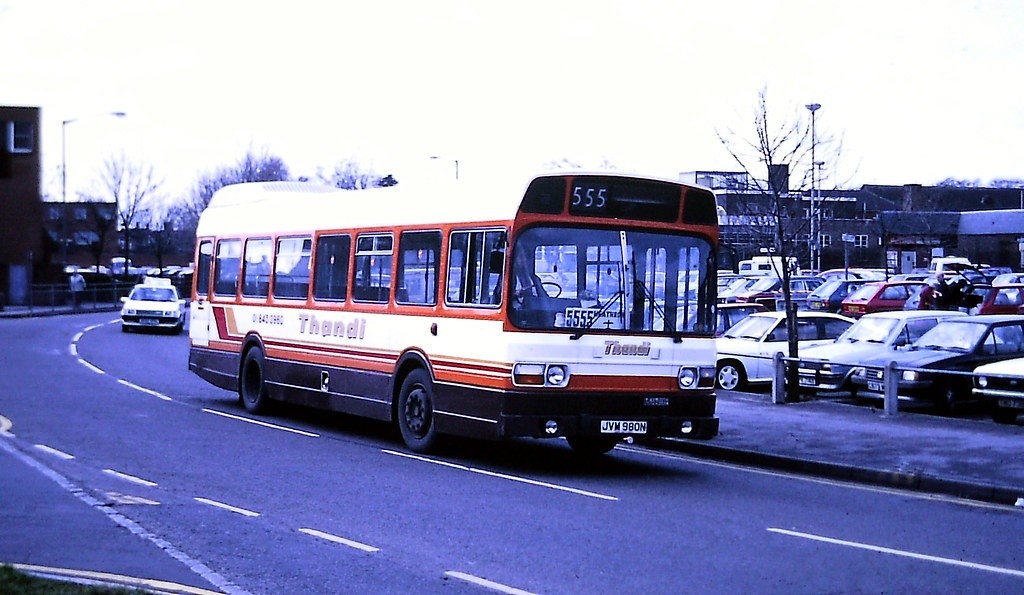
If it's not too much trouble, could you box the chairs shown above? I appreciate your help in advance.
[218,273,408,300]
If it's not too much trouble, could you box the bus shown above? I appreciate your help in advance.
[188,174,738,453]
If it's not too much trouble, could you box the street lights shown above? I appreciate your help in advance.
[61,111,125,201]
[430,156,458,178]
[814,161,825,271]
[806,104,822,274]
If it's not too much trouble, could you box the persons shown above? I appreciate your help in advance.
[918,273,950,310]
[949,278,967,311]
[70,269,86,307]
[493,244,548,307]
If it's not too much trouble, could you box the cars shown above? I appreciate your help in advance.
[783,310,1003,396]
[602,300,859,390]
[65,257,194,275]
[717,256,1024,320]
[850,315,1024,419]
[973,357,1024,422]
[120,278,186,335]
[370,269,702,300]
